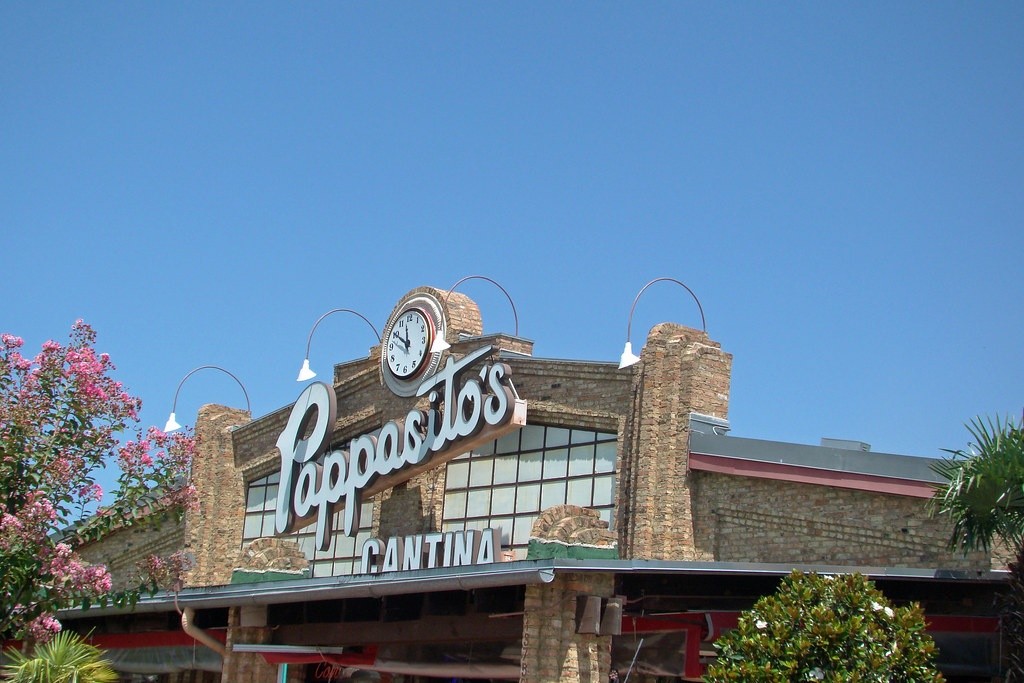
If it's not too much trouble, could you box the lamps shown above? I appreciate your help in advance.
[617,277,708,372]
[297,308,384,382]
[431,275,520,355]
[164,366,250,433]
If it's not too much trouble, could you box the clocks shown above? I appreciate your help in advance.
[384,307,437,381]
